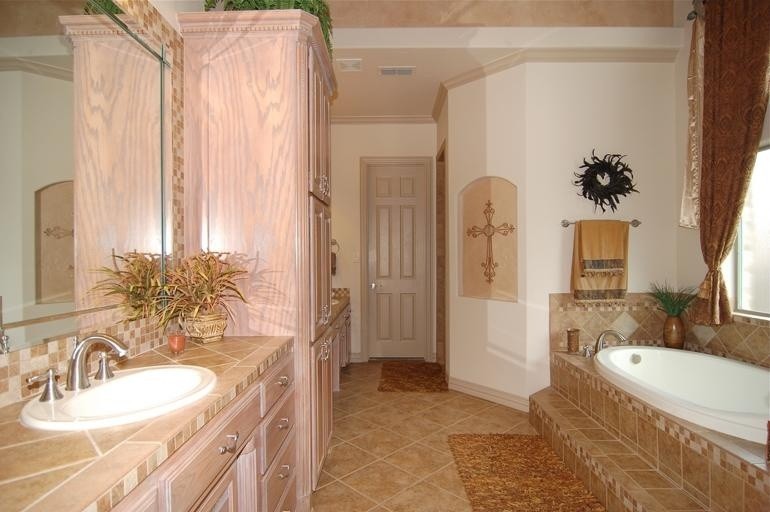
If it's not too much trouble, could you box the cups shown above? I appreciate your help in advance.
[168,329,186,355]
[566,328,580,355]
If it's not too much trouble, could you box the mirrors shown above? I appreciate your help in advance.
[0,0,174,354]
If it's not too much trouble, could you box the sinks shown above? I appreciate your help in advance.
[19,364,217,433]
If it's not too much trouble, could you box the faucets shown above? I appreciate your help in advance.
[594,330,627,353]
[65,332,130,391]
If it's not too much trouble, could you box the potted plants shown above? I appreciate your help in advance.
[647,279,700,350]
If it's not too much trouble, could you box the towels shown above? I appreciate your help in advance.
[571,220,629,306]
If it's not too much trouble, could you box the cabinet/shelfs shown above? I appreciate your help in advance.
[332,297,350,390]
[110,336,296,512]
[177,9,334,511]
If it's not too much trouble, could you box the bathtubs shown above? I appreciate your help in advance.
[593,345,769,445]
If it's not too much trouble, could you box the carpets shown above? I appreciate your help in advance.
[378,361,448,392]
[448,434,606,512]
